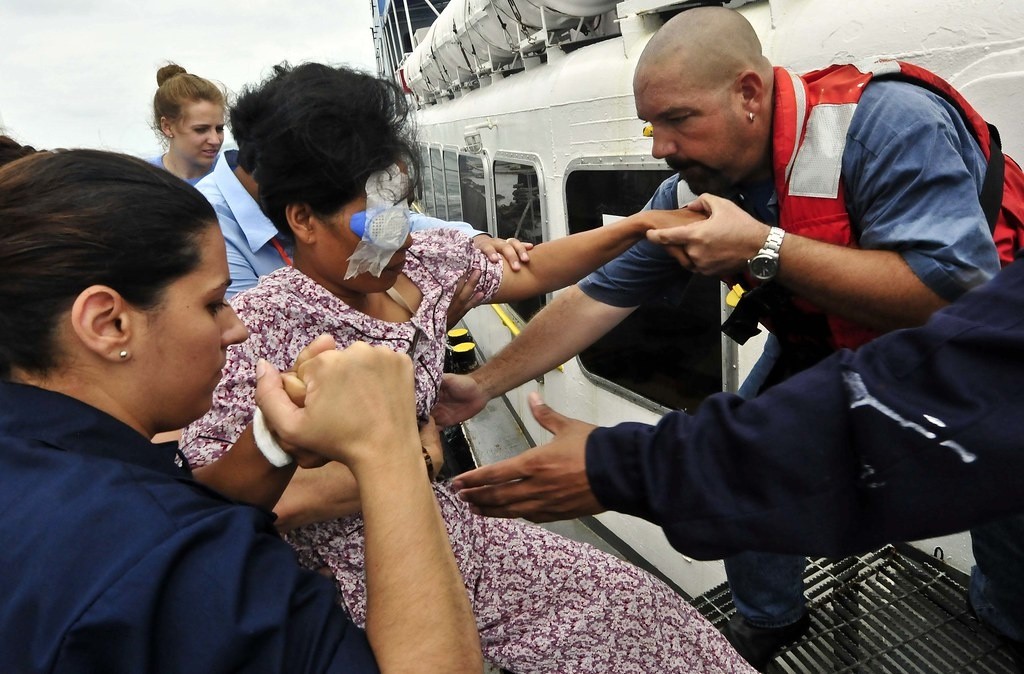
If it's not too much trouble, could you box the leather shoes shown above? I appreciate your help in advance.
[720,611,811,672]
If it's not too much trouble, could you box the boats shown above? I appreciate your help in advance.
[368,0,1024,672]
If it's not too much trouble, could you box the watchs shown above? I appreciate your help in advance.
[747,227,786,284]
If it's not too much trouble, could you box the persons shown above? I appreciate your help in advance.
[430,7,1024,658]
[449,249,1024,665]
[142,66,230,189]
[0,147,481,674]
[178,63,750,670]
[193,144,535,301]
